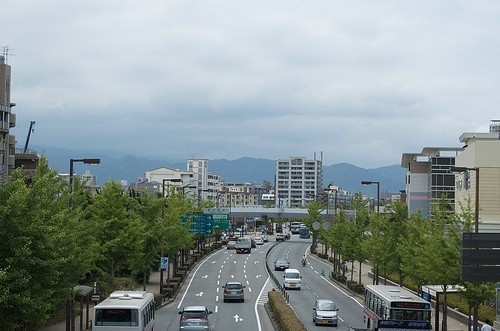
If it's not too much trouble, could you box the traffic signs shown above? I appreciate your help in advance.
[182,213,214,234]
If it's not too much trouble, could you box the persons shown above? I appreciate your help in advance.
[480,319,491,331]
[219,232,226,240]
[301,255,306,263]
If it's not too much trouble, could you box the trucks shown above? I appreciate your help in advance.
[235,237,253,254]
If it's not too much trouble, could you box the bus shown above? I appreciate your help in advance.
[291,222,310,238]
[92,290,156,331]
[362,284,432,331]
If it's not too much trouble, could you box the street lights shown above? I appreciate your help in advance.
[450,166,480,331]
[324,189,341,282]
[159,178,182,293]
[361,181,380,285]
[66,158,101,331]
[317,191,328,259]
[182,184,197,267]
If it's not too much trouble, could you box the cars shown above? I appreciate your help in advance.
[276,232,292,242]
[222,282,246,303]
[312,300,339,327]
[178,305,213,331]
[274,258,290,271]
[251,234,269,248]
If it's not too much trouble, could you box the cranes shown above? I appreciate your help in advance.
[23,120,36,154]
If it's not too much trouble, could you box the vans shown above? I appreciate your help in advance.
[283,268,302,290]
[227,240,236,250]
